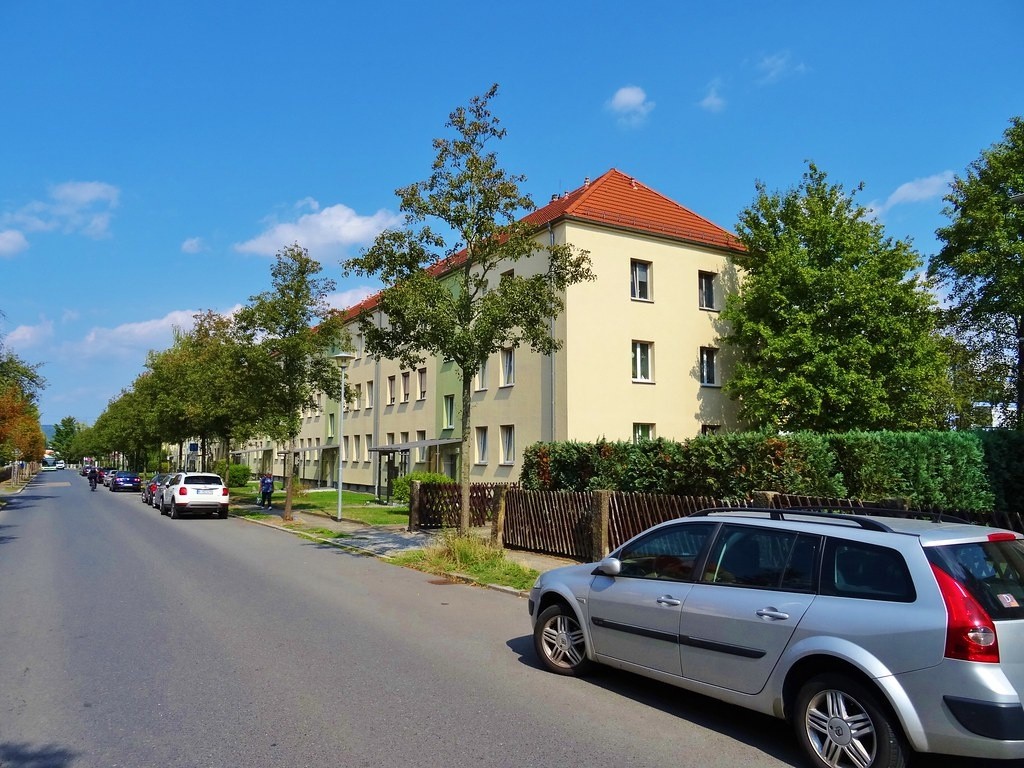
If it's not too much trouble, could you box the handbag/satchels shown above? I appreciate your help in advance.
[256,497,262,504]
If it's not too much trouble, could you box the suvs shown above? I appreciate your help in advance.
[159,471,229,519]
[528,505,1024,768]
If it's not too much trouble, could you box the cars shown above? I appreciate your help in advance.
[102,470,117,486]
[82,464,116,484]
[109,471,141,492]
[142,474,159,503]
[152,474,174,509]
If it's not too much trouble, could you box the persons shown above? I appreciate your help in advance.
[87,466,97,489]
[259,471,274,511]
[662,550,691,579]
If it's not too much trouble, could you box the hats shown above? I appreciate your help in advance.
[91,466,95,468]
[267,471,272,474]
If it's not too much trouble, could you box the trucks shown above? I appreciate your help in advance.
[56,460,66,470]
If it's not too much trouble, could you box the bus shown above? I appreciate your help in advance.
[41,457,57,472]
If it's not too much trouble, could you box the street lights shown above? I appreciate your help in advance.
[328,351,355,522]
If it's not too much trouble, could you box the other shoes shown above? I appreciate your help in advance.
[267,507,272,510]
[260,507,264,509]
